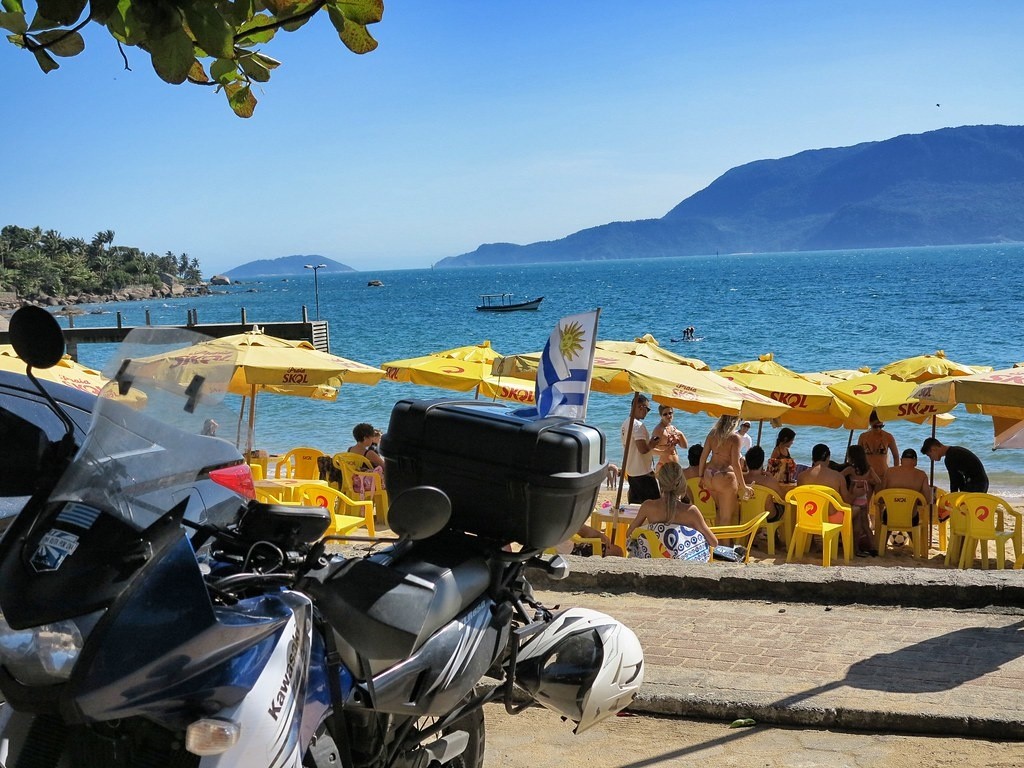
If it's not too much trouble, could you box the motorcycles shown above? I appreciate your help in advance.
[1,298,646,768]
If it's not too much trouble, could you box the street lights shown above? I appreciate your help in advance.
[304,265,328,320]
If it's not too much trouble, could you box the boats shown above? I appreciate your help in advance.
[475,293,544,310]
[367,280,382,288]
[669,336,706,342]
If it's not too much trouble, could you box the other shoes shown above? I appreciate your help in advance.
[855,548,867,557]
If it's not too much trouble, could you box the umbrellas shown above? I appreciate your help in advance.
[0,325,1024,546]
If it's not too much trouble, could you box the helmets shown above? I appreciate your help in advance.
[502,607,645,734]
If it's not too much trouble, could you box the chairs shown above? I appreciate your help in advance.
[237,444,1024,573]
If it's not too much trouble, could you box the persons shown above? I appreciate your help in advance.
[683,329,686,339]
[347,422,385,473]
[566,395,990,562]
[368,429,383,456]
[200,419,218,437]
[686,327,691,340]
[690,326,694,339]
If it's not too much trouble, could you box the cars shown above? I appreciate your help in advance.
[0,369,257,555]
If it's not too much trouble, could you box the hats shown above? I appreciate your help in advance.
[741,421,751,426]
[902,449,917,458]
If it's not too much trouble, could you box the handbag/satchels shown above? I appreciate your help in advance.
[352,466,385,516]
[767,457,795,481]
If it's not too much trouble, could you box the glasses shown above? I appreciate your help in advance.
[743,424,750,428]
[663,412,673,416]
[873,424,885,429]
[641,405,651,412]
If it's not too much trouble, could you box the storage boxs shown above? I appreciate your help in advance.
[380,398,608,554]
